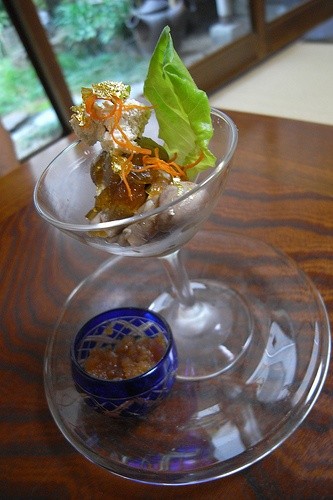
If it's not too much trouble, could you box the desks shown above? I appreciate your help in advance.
[0,106,333,500]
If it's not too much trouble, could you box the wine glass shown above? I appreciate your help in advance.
[32,105,331,486]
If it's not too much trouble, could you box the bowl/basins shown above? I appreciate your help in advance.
[70,307,178,418]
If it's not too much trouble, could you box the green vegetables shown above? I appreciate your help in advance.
[142,26,218,182]
